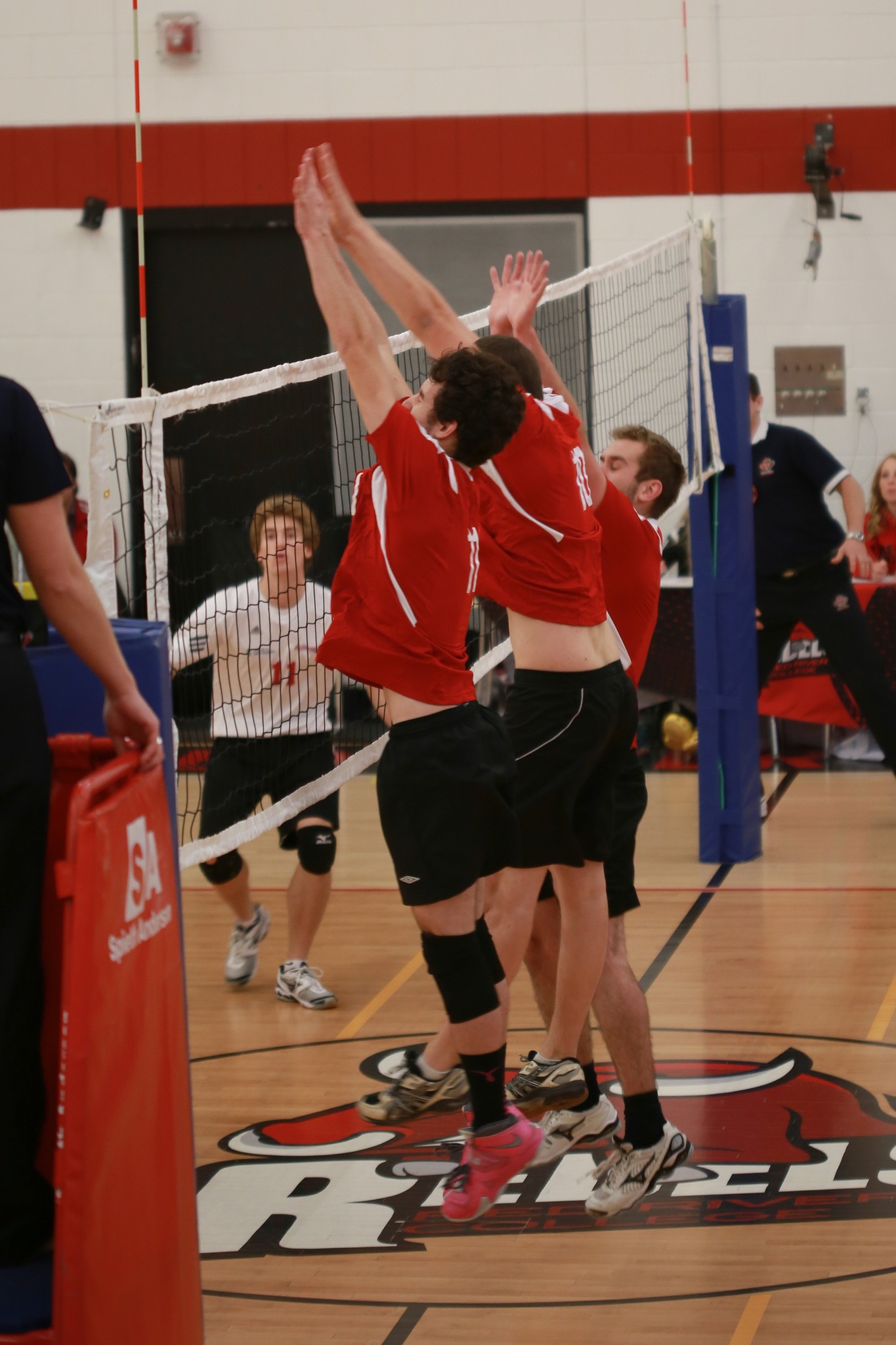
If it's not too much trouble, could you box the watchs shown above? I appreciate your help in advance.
[846,531,866,542]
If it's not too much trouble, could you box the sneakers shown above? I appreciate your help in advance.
[462,1051,589,1118]
[583,1120,694,1219]
[275,962,338,1009]
[526,1093,622,1168]
[357,1049,471,1126]
[441,1097,546,1223]
[223,902,271,988]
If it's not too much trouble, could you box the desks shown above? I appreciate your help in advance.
[639,577,896,761]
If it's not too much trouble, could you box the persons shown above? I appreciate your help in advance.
[293,142,895,1225]
[0,377,163,1262]
[166,494,342,1008]
